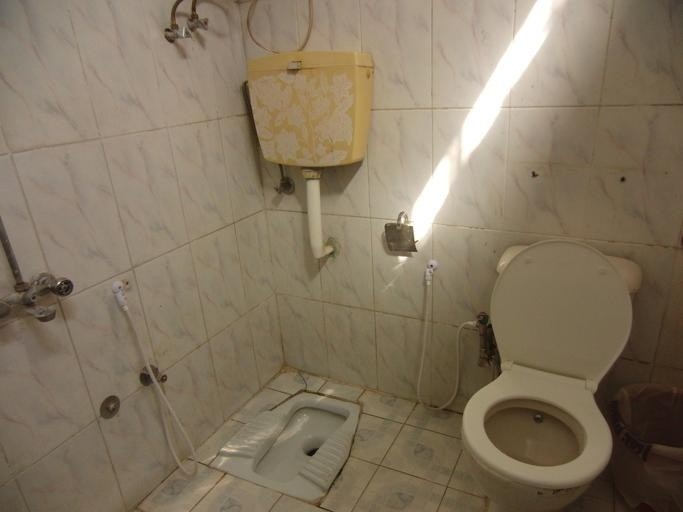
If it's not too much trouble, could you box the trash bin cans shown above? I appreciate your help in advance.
[608,382,683,512]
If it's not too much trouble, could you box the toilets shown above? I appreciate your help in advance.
[458,236,645,512]
